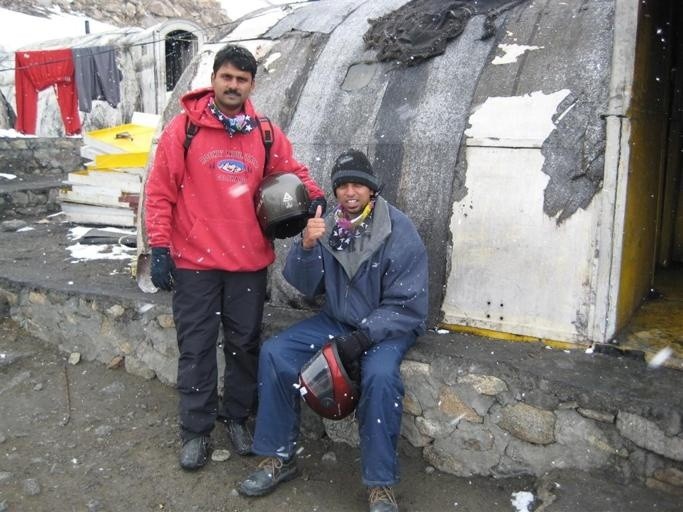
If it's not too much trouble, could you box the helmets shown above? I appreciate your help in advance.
[299,343,360,420]
[254,171,311,237]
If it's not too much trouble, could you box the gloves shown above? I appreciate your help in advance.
[151,248,178,292]
[336,330,371,362]
[309,196,326,218]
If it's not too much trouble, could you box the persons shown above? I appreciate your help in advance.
[143,44,326,472]
[237,148,430,512]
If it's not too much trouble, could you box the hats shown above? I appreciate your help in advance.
[331,148,378,198]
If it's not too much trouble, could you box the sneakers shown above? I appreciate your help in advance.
[225,419,255,454]
[239,456,299,496]
[368,485,399,512]
[180,432,211,467]
